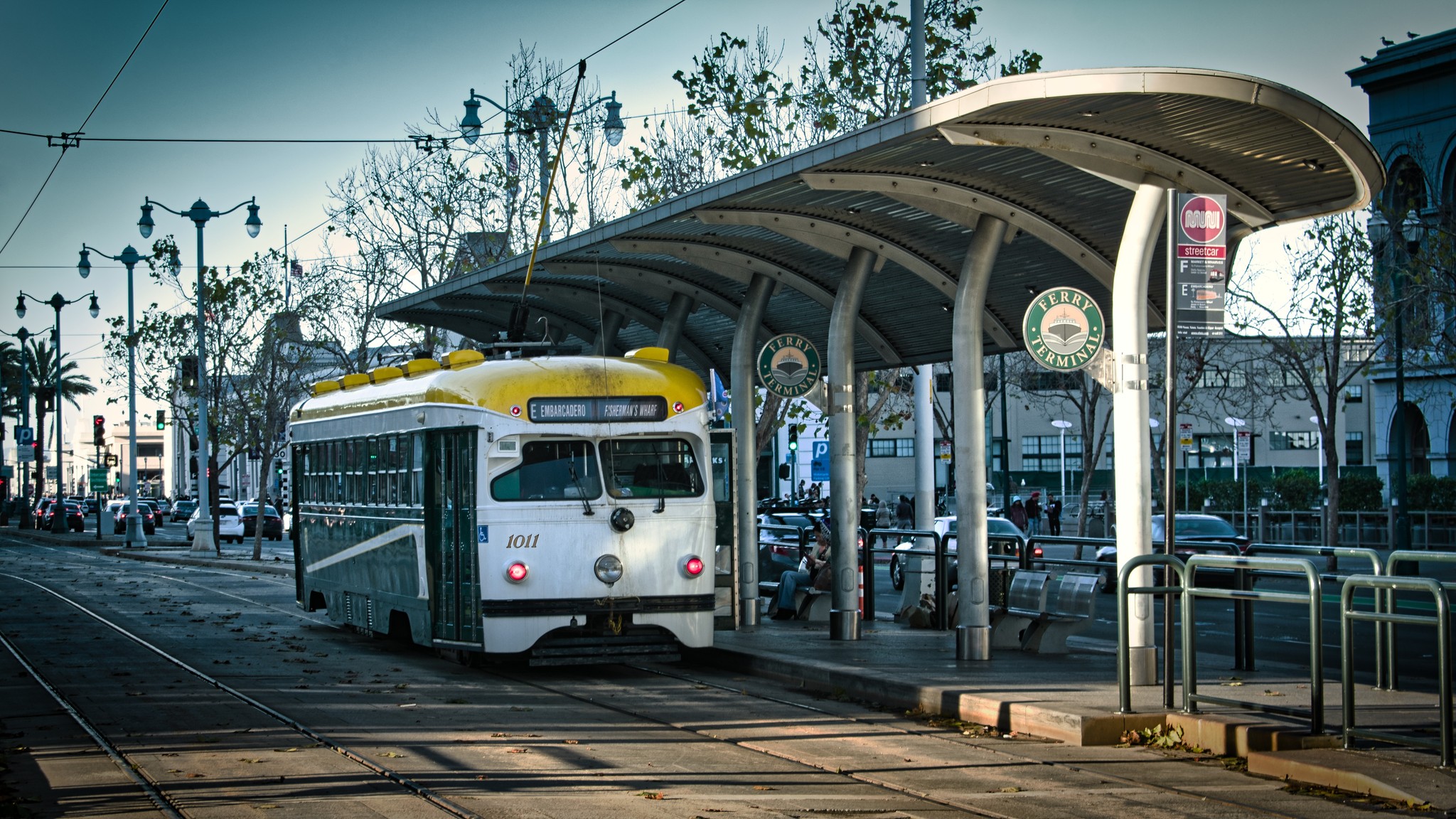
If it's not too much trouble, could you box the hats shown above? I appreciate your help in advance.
[1031,491,1040,498]
[1013,496,1021,503]
[986,483,995,490]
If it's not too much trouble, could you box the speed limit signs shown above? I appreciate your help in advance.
[272,442,287,459]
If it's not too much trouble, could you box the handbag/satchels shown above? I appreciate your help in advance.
[814,563,832,591]
[810,554,826,579]
[889,511,893,520]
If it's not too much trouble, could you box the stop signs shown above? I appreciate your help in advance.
[28,485,35,495]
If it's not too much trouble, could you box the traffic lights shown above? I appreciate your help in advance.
[93,415,105,446]
[275,461,283,474]
[156,410,166,430]
[788,424,798,451]
[115,472,120,482]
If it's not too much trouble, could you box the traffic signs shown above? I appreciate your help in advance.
[89,468,108,492]
[1180,428,1192,451]
[940,442,951,464]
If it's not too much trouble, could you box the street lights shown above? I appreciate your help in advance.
[460,87,626,244]
[1,289,101,534]
[1052,420,1072,507]
[158,454,163,500]
[76,242,185,547]
[144,457,148,497]
[119,458,123,498]
[1224,416,1246,483]
[1366,210,1424,577]
[1309,416,1328,486]
[136,196,264,558]
[177,452,182,500]
[66,463,96,499]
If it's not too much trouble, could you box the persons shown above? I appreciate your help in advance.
[1010,495,1029,536]
[1042,493,1063,535]
[861,493,879,507]
[785,479,830,508]
[1099,490,1108,502]
[273,495,284,522]
[1025,492,1041,538]
[875,500,892,550]
[892,493,940,549]
[771,519,831,620]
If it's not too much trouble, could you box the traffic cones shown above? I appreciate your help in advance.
[858,566,864,620]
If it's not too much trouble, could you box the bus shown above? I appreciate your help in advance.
[284,59,740,674]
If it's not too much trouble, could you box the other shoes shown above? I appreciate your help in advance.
[883,545,887,548]
[893,546,897,548]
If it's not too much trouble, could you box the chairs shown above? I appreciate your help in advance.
[984,569,1106,650]
[759,556,832,595]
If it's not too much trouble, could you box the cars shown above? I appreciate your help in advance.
[889,516,1046,606]
[0,494,293,544]
[756,497,865,583]
[1094,514,1257,600]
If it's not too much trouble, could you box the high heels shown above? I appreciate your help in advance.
[771,608,797,621]
